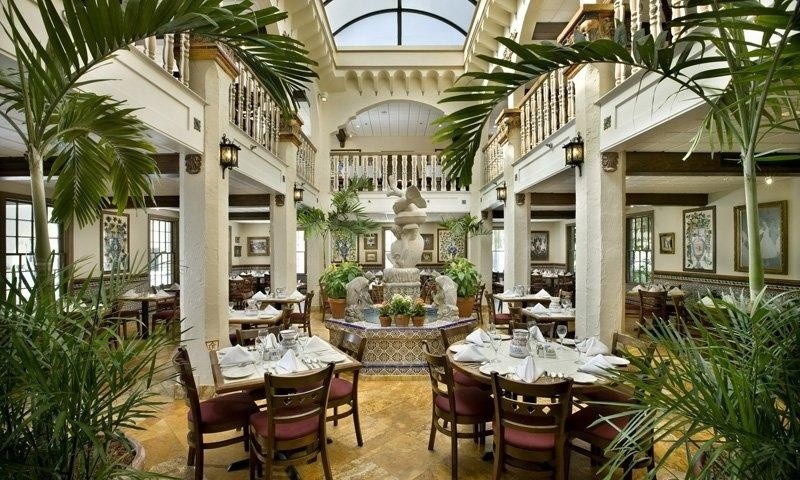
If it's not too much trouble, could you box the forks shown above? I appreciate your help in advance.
[302,357,322,369]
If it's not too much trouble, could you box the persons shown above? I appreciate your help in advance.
[758,221,779,259]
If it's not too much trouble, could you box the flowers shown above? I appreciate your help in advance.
[378,294,426,317]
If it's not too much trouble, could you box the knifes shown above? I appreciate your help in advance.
[275,363,288,372]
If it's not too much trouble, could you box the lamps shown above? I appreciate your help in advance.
[318,92,329,102]
[294,182,304,202]
[496,181,507,206]
[220,134,241,178]
[562,132,584,176]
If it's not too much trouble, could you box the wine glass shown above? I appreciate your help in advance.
[253,328,271,368]
[264,286,292,300]
[489,334,503,364]
[526,320,538,347]
[561,299,571,313]
[555,324,568,352]
[297,331,309,356]
[486,324,496,349]
[572,335,587,365]
[227,298,266,320]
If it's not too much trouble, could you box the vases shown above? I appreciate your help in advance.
[379,313,425,327]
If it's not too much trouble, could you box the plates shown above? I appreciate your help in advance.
[221,368,255,379]
[449,344,470,353]
[219,347,236,354]
[478,362,513,376]
[567,372,598,384]
[602,355,632,366]
[556,339,574,345]
[492,334,511,340]
[318,354,345,364]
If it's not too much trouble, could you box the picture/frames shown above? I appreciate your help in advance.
[100,211,130,274]
[531,231,549,261]
[437,228,466,262]
[420,234,434,250]
[247,237,270,256]
[421,252,432,262]
[682,205,716,273]
[734,200,788,275]
[330,229,378,263]
[659,232,675,254]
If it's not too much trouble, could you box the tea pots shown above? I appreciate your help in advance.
[277,329,299,358]
[509,328,530,358]
[548,296,561,314]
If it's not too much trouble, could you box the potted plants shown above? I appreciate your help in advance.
[584,287,800,480]
[320,262,364,319]
[0,246,198,480]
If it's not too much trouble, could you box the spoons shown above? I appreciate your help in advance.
[263,362,279,376]
[543,368,564,385]
